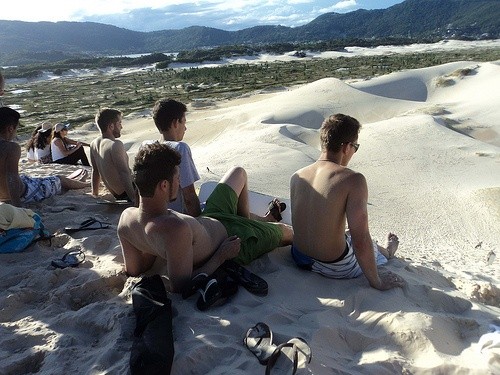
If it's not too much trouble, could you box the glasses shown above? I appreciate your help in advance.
[341,142,360,153]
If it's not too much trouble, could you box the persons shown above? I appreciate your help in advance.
[26,120,92,166]
[139,97,286,222]
[0,107,92,209]
[117,143,295,294]
[86,109,139,206]
[290,113,405,291]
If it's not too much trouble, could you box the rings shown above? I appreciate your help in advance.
[395,277,397,282]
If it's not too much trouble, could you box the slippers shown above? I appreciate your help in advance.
[265,337,313,375]
[65,218,109,232]
[180,259,269,312]
[51,249,85,269]
[243,321,278,365]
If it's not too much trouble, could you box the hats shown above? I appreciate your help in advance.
[55,123,70,133]
[38,122,53,132]
